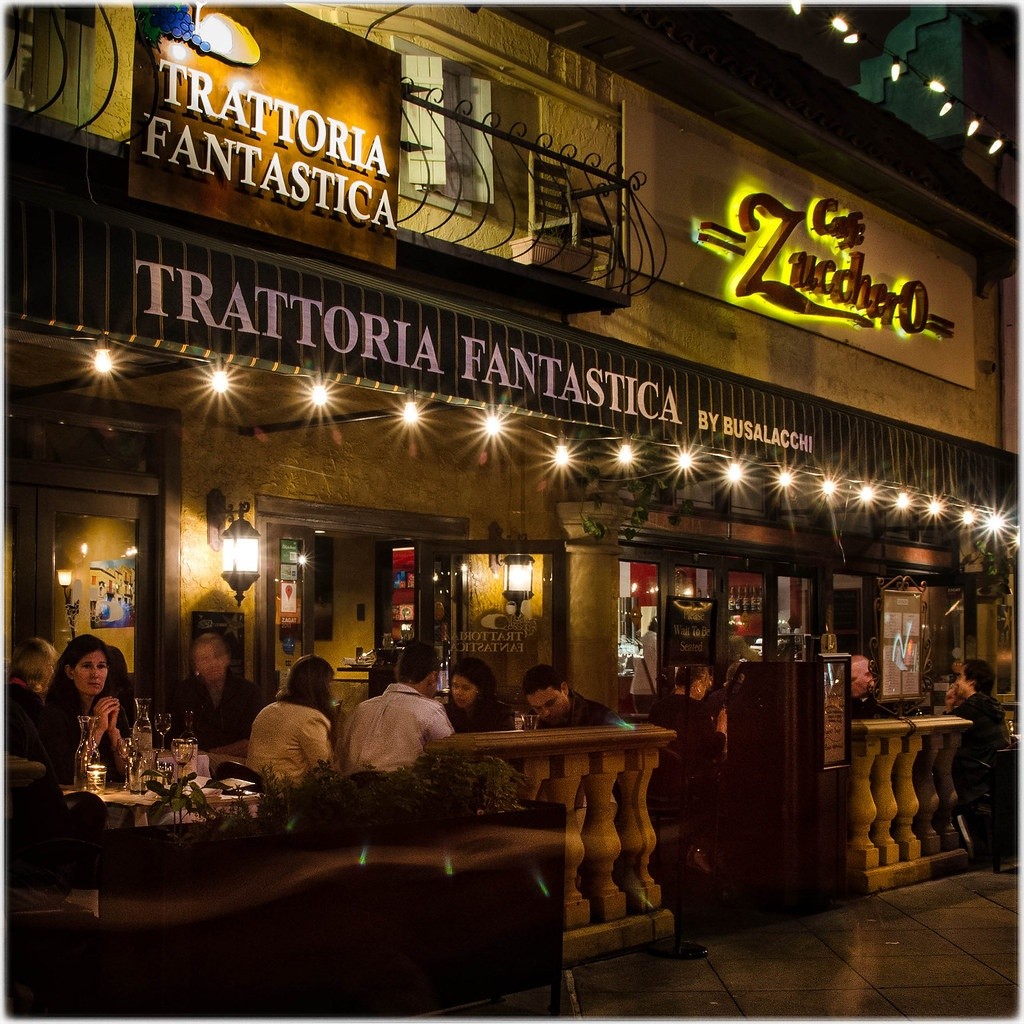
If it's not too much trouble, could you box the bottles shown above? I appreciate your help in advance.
[383,633,394,647]
[133,698,152,752]
[728,587,736,615]
[434,692,441,702]
[778,620,790,634]
[743,585,750,612]
[778,638,803,660]
[443,691,449,704]
[736,586,742,615]
[180,712,197,776]
[74,716,99,790]
[757,586,764,612]
[751,586,757,611]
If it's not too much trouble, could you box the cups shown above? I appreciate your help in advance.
[521,715,539,730]
[86,765,106,793]
[128,752,150,793]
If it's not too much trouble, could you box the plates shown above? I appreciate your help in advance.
[349,664,371,668]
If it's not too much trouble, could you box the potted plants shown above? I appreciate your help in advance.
[509,215,598,280]
[98,731,567,1016]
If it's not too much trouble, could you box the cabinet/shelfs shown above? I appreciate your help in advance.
[393,588,447,624]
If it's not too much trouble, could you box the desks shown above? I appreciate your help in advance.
[60,784,272,831]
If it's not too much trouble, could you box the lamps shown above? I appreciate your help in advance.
[207,486,261,609]
[489,520,533,618]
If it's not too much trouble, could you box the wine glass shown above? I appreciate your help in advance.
[117,739,137,790]
[154,712,172,753]
[171,739,194,781]
[152,749,174,786]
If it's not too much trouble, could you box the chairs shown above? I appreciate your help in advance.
[528,149,620,290]
[212,759,268,796]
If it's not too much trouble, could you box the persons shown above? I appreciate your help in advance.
[159,632,269,760]
[245,654,335,789]
[646,664,727,874]
[521,663,625,730]
[851,654,888,719]
[727,621,762,664]
[6,635,138,783]
[441,658,515,734]
[630,616,658,714]
[335,640,455,772]
[941,659,1009,783]
